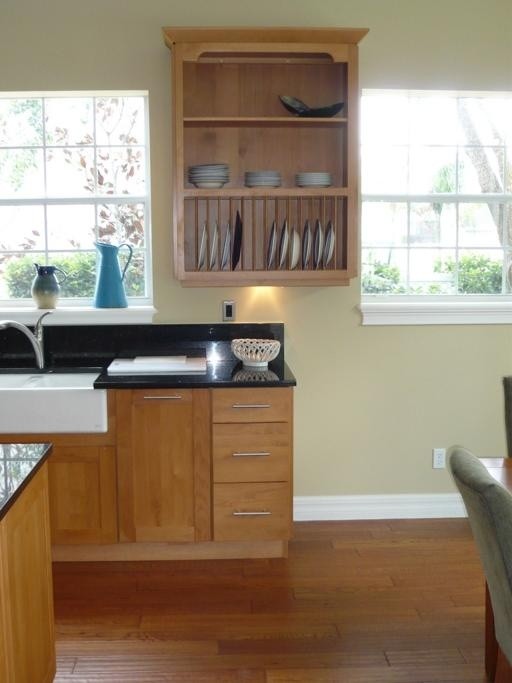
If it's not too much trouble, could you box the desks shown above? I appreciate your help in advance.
[474,454,512,682]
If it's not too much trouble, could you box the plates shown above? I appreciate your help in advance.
[314,217,323,269]
[209,221,219,270]
[303,218,313,269]
[232,207,242,270]
[196,222,208,271]
[220,221,231,270]
[278,219,288,269]
[324,218,334,269]
[266,219,276,270]
[184,163,337,190]
[289,227,301,269]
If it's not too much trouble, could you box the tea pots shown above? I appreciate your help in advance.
[30,262,68,311]
[91,240,134,309]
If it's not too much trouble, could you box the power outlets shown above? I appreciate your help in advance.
[433,447,446,470]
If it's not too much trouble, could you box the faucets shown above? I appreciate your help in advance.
[0,309,53,371]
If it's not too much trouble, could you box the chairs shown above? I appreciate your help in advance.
[503,377,512,458]
[449,445,512,683]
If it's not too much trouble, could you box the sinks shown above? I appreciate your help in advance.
[31,371,108,434]
[0,368,32,434]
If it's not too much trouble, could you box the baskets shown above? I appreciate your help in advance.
[231,338,281,367]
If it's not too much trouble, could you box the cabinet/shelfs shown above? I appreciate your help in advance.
[2,443,59,681]
[119,390,213,560]
[2,390,118,562]
[161,25,370,288]
[214,387,294,560]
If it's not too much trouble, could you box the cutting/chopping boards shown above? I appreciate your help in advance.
[106,356,208,375]
